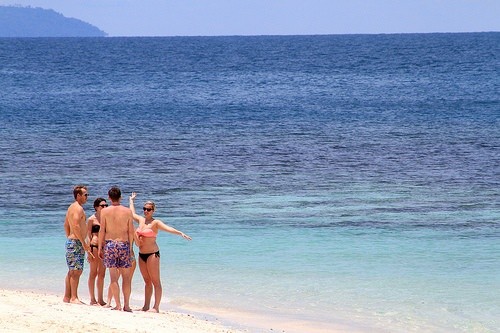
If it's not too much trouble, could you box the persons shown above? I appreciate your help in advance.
[63,186,92,305]
[87,198,112,307]
[129,191,192,313]
[98,187,134,314]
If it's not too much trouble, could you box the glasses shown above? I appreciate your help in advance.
[143,207,154,211]
[99,204,108,208]
[82,194,89,196]
